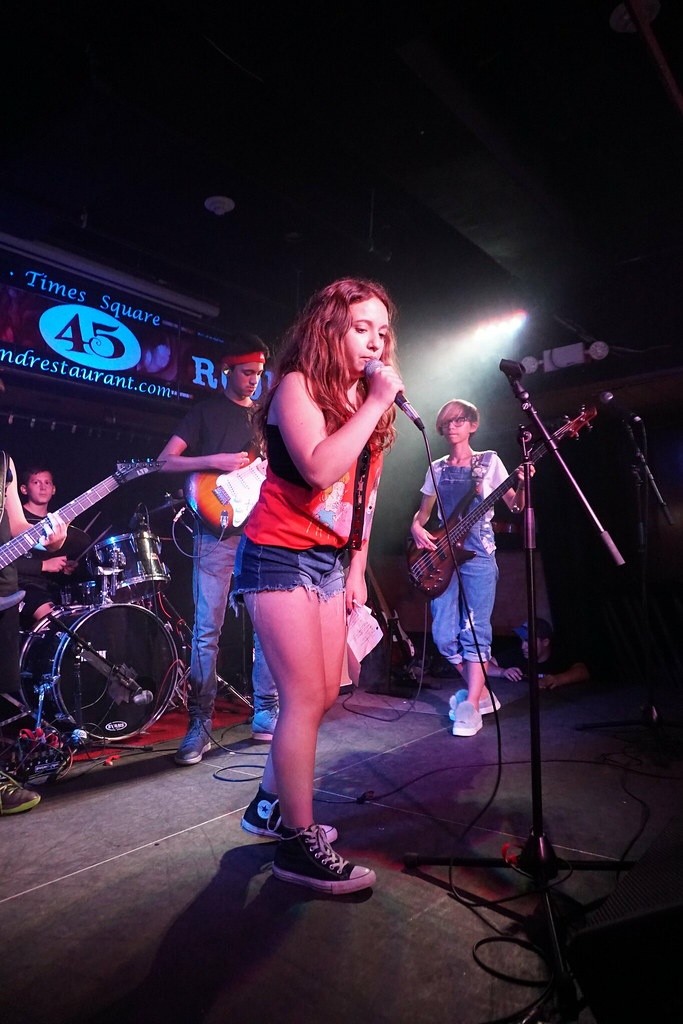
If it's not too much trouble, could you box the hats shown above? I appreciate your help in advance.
[512,618,552,639]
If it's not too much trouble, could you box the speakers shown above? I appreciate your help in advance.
[216,601,256,700]
[571,807,683,1024]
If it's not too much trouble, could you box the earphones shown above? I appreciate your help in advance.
[223,369,230,374]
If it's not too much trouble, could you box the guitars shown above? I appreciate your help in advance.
[403,390,615,600]
[0,459,167,612]
[365,560,416,678]
[183,438,268,540]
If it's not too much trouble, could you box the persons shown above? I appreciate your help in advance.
[493,618,590,685]
[0,450,67,816]
[155,330,279,766]
[228,276,404,896]
[410,400,536,736]
[10,463,78,621]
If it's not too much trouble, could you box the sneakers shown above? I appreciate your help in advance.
[272,821,376,894]
[241,781,337,843]
[453,701,482,736]
[252,706,279,740]
[0,771,41,815]
[449,689,501,720]
[174,718,212,764]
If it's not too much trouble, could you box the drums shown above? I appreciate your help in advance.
[18,603,179,741]
[60,580,103,606]
[85,532,172,604]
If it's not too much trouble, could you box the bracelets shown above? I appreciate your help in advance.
[518,485,525,491]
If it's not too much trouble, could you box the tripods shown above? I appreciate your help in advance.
[42,614,154,783]
[403,358,683,1024]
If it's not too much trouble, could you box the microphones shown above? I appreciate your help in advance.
[133,689,153,706]
[130,505,141,529]
[364,359,425,432]
[599,392,642,424]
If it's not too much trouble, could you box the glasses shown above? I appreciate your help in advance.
[439,416,472,427]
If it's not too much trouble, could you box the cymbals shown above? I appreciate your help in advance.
[25,517,92,557]
[160,536,179,541]
[144,498,185,516]
